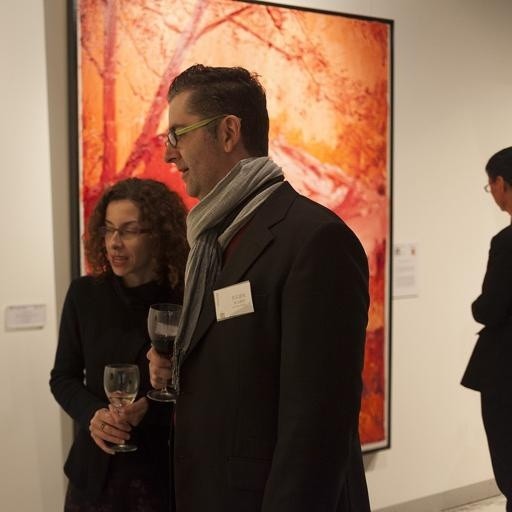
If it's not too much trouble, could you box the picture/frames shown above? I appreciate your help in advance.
[67,1,393,458]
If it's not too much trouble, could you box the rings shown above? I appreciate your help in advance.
[100,423,106,431]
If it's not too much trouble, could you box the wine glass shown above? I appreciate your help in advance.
[142,303,184,404]
[103,361,143,457]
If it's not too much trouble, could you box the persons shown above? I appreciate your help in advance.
[49,175,190,512]
[146,63,371,512]
[460,144,512,512]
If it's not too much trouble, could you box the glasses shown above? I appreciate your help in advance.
[165,114,242,149]
[484,181,495,192]
[96,225,150,239]
